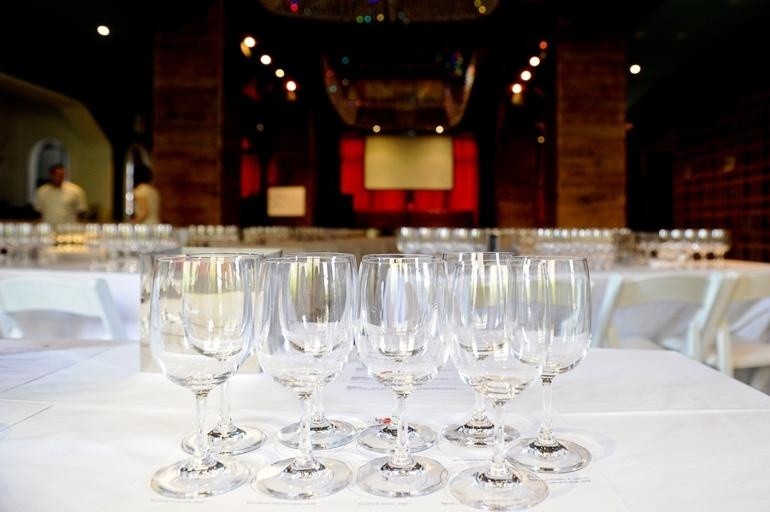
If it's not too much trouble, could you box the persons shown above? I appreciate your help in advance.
[31,164,90,223]
[125,163,161,224]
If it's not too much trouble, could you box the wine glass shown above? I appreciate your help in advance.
[396,226,732,270]
[148,251,593,510]
[0,222,178,272]
